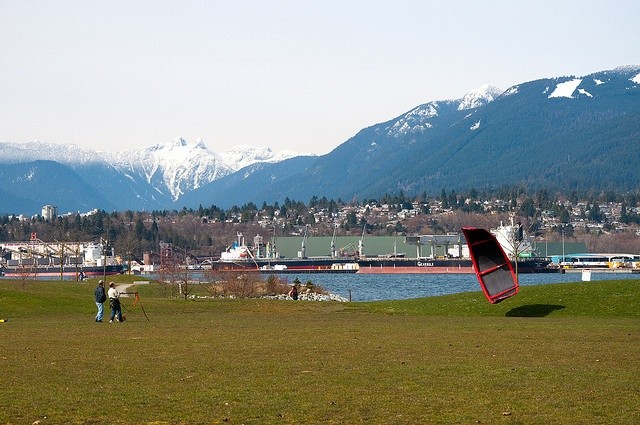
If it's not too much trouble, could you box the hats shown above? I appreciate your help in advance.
[97,280,105,284]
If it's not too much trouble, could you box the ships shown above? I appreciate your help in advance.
[358,257,552,274]
[3,265,123,277]
[199,234,359,274]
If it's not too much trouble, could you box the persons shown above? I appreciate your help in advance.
[78,272,83,280]
[94,280,107,322]
[107,282,126,324]
[83,272,86,278]
[289,286,298,300]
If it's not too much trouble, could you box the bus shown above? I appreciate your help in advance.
[560,253,609,268]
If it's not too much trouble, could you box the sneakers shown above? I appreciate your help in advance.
[94,318,98,322]
[108,320,113,323]
[98,320,104,323]
